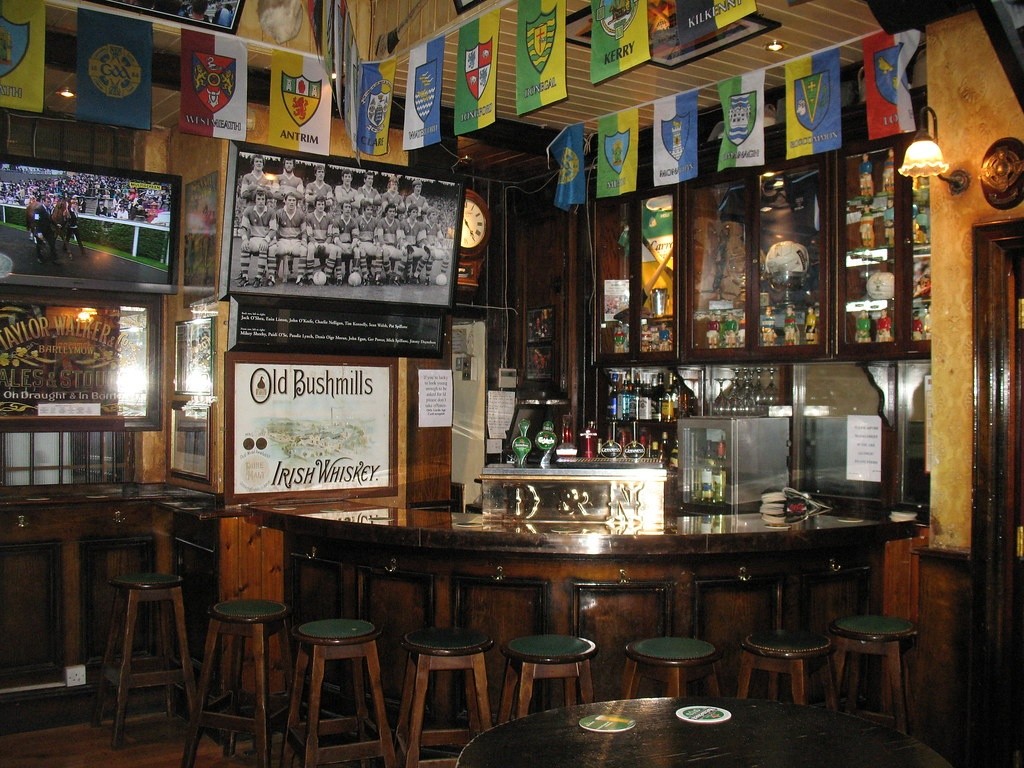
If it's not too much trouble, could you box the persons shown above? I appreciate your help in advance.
[856,309,892,342]
[912,311,931,341]
[236,154,450,288]
[614,325,626,354]
[641,321,672,352]
[0,173,171,224]
[706,309,746,349]
[761,306,816,346]
[859,148,894,246]
[26,196,88,263]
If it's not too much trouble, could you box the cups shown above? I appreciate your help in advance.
[652,288,665,316]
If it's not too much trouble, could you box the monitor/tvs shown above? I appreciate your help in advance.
[0,153,182,295]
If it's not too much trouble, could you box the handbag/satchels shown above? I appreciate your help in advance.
[758,486,832,524]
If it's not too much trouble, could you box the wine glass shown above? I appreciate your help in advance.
[712,367,779,416]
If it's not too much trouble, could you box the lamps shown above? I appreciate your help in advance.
[898,106,971,196]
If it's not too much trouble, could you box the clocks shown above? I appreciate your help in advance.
[456,189,492,294]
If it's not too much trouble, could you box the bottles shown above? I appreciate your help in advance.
[609,372,726,503]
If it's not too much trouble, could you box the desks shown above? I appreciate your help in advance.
[455,697,953,768]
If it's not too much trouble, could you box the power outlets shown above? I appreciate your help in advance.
[64,664,86,687]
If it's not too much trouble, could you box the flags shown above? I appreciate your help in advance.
[0,0,46,113]
[402,35,441,150]
[454,8,500,135]
[549,29,920,211]
[74,4,153,131]
[268,48,332,156]
[178,28,247,142]
[516,0,567,116]
[356,57,397,156]
[590,0,651,85]
[675,0,757,45]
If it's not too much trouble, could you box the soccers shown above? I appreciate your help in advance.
[313,271,326,285]
[436,274,447,286]
[348,272,361,286]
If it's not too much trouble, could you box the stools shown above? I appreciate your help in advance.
[89,572,198,750]
[279,618,399,768]
[497,633,598,725]
[393,626,493,768]
[181,598,294,768]
[830,615,919,741]
[736,628,839,712]
[617,635,721,700]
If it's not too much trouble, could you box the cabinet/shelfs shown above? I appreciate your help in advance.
[582,84,931,455]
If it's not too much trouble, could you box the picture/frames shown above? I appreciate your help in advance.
[166,399,224,494]
[565,6,783,72]
[220,141,467,316]
[173,316,217,397]
[524,305,556,383]
